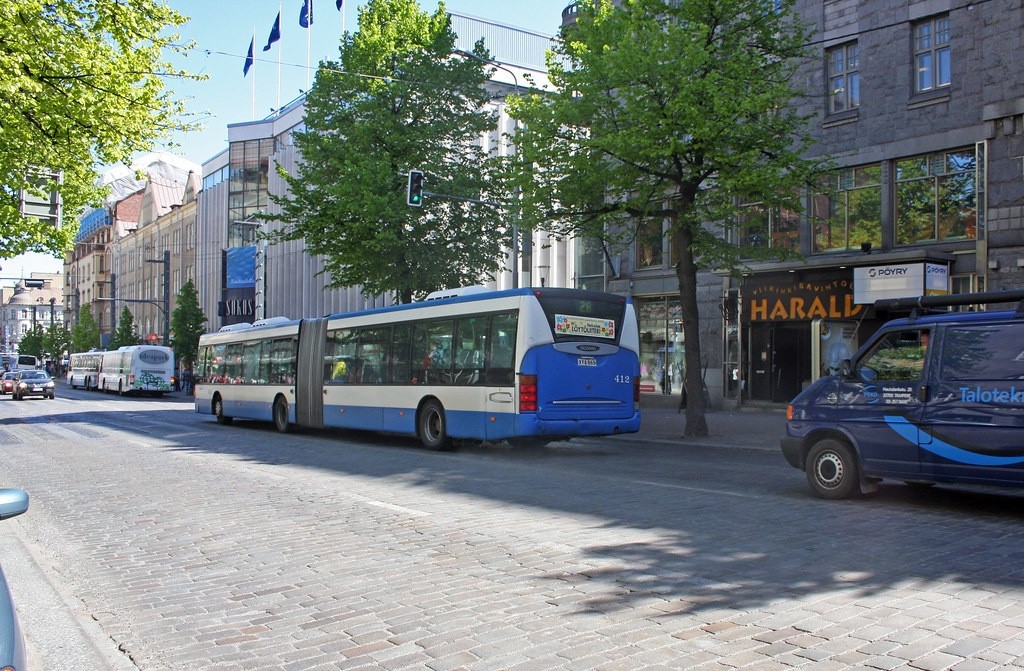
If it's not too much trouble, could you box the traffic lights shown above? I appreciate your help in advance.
[406,169,424,206]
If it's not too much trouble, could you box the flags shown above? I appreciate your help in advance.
[243,36,253,77]
[299,0,313,28]
[263,11,280,51]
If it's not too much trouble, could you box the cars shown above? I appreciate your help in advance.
[0,345,56,401]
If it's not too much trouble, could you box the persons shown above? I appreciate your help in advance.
[35,361,69,379]
[174,367,191,395]
[889,329,930,381]
[333,328,514,387]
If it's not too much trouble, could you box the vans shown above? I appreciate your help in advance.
[778,286,1024,504]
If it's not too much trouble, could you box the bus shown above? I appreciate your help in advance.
[194,283,643,453]
[65,344,175,396]
